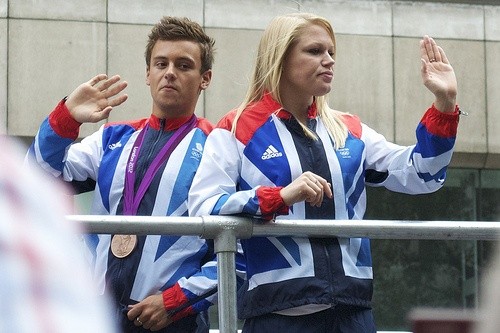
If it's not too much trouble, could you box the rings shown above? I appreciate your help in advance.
[430,57,435,61]
[136,317,142,325]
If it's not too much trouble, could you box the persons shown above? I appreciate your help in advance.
[188,12,461,333]
[20,15,247,333]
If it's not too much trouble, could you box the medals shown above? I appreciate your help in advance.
[111,234,136,259]
